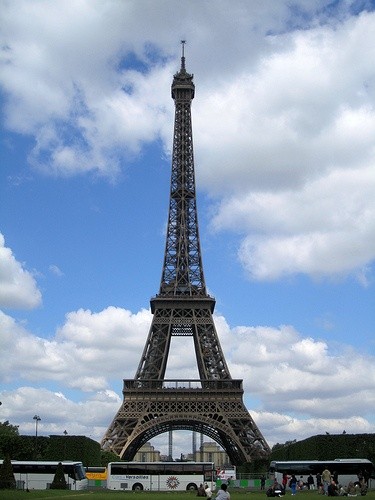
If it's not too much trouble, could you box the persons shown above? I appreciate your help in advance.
[197,476,232,500]
[261,466,369,497]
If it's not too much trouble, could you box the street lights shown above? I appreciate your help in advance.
[63,430,68,461]
[33,414,41,459]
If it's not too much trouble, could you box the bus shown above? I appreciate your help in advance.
[269,459,375,490]
[216,465,236,481]
[0,459,89,492]
[106,461,218,492]
[83,467,107,480]
